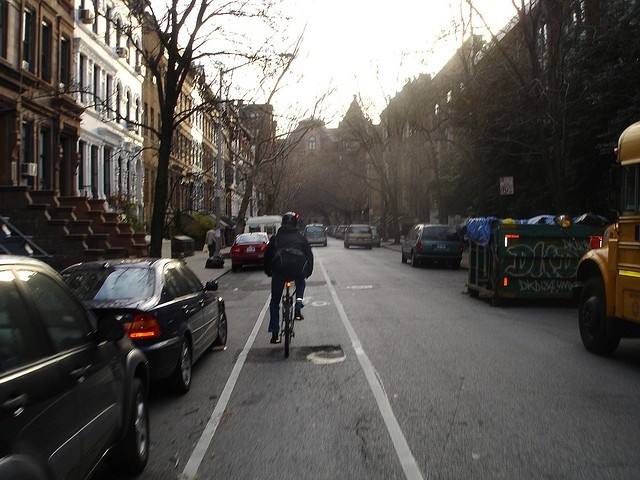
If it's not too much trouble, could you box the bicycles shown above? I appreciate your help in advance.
[267,272,308,358]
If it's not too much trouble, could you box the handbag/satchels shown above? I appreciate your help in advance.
[272,248,309,280]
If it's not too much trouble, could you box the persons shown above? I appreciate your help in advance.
[263,211,314,345]
[206,226,216,258]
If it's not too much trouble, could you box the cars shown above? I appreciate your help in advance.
[326,223,382,250]
[231,231,270,272]
[60,256,228,393]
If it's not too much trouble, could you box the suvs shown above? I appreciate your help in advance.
[1,250,152,480]
[401,223,465,270]
[305,224,328,247]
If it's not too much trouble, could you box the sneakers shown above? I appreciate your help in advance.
[270,337,277,343]
[295,312,304,319]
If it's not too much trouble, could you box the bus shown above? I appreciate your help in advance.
[574,121,639,354]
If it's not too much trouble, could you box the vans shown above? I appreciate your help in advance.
[243,215,281,239]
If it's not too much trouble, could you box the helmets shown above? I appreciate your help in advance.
[282,213,298,226]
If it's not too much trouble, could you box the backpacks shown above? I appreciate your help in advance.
[270,235,310,276]
